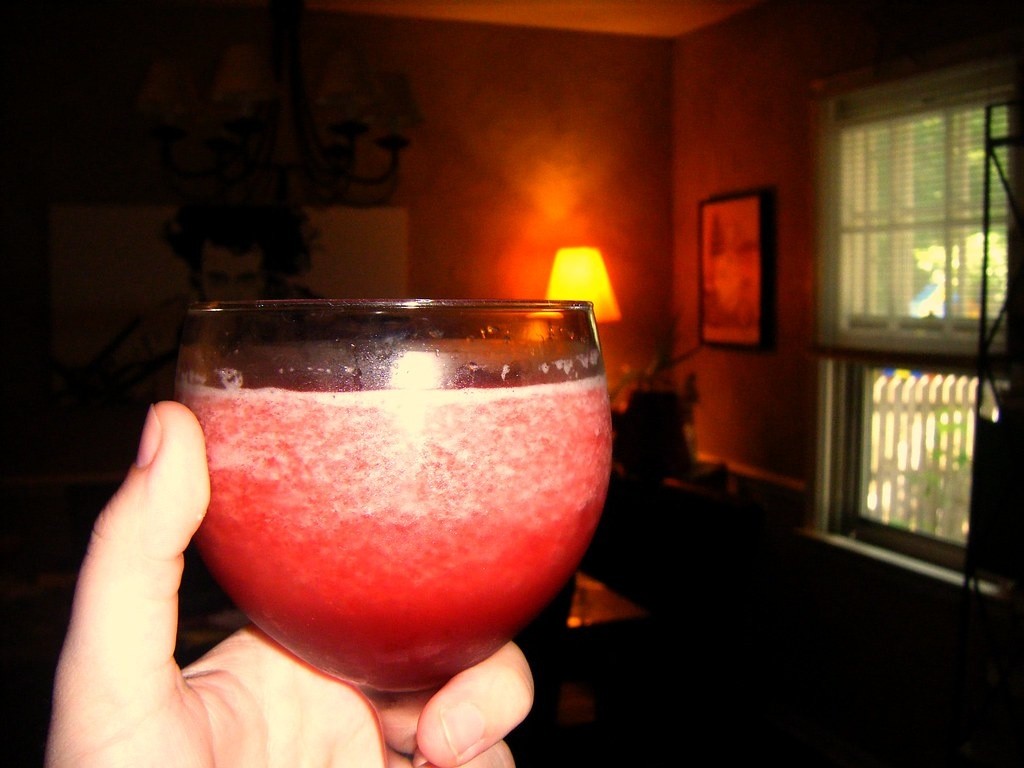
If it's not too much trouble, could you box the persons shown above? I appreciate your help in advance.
[46,400,533,768]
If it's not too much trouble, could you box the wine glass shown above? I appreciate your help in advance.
[175,298,612,768]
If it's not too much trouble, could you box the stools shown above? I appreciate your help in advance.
[562,571,652,722]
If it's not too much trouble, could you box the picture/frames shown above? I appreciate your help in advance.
[697,188,777,353]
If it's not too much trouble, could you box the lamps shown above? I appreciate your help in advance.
[545,245,622,322]
[134,0,421,296]
